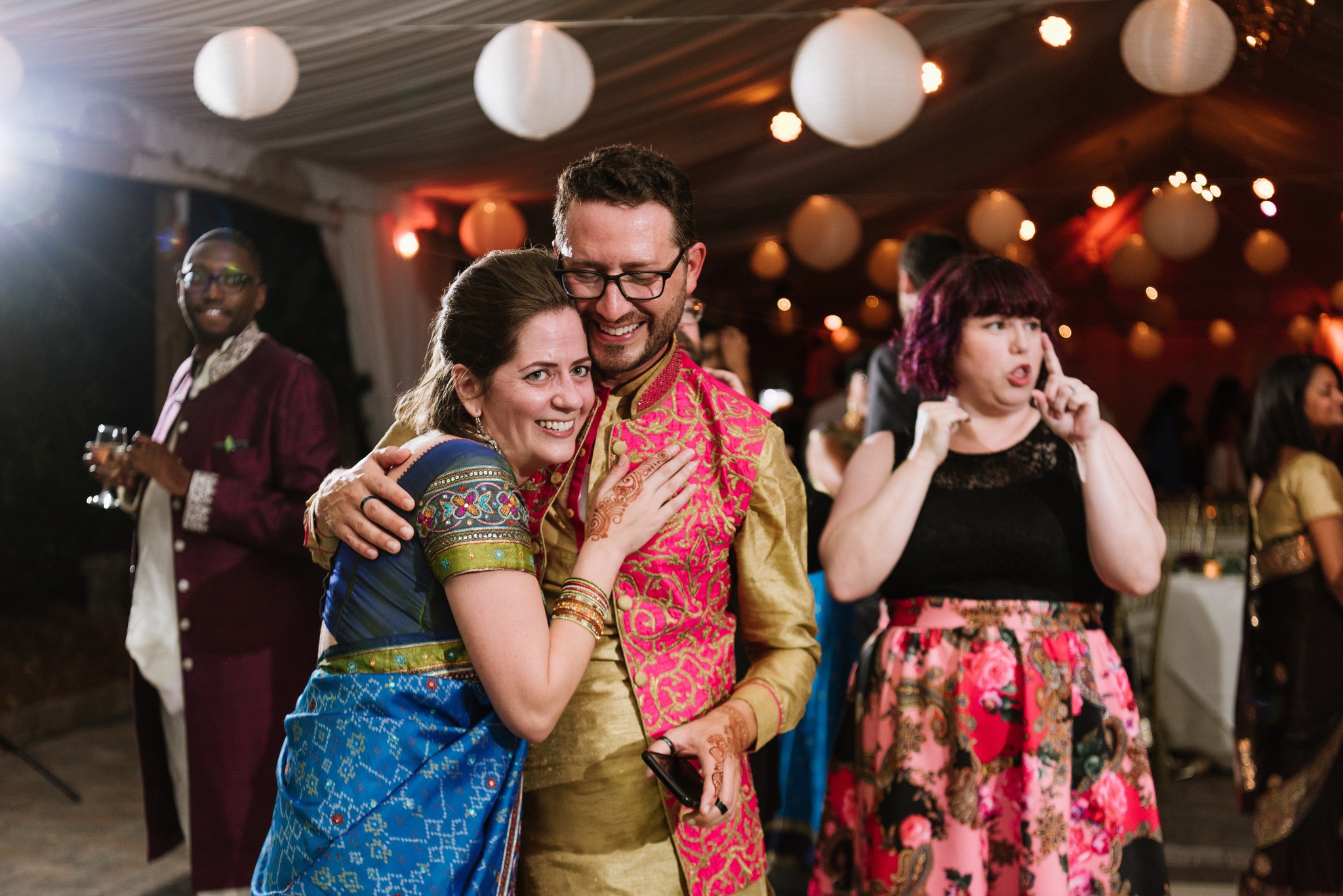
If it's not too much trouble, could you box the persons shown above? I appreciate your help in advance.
[299,143,822,896]
[813,254,1174,896]
[761,351,858,895]
[859,224,990,441]
[1232,351,1342,895]
[80,228,342,896]
[232,246,703,895]
[1133,373,1255,508]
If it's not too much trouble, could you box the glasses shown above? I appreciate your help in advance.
[552,240,693,299]
[176,267,260,296]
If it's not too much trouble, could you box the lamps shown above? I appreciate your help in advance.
[471,20,595,138]
[461,177,1343,364]
[1121,0,1237,98]
[787,8,928,151]
[192,28,300,120]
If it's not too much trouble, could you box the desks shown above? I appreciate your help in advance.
[1158,568,1243,779]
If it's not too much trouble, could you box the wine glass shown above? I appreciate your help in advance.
[87,423,129,509]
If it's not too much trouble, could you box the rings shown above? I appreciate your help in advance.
[360,496,377,515]
[655,736,676,756]
[714,797,727,816]
[1067,384,1077,396]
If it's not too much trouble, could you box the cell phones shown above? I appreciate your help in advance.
[642,747,706,810]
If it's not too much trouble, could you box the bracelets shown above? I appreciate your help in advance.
[549,576,610,641]
